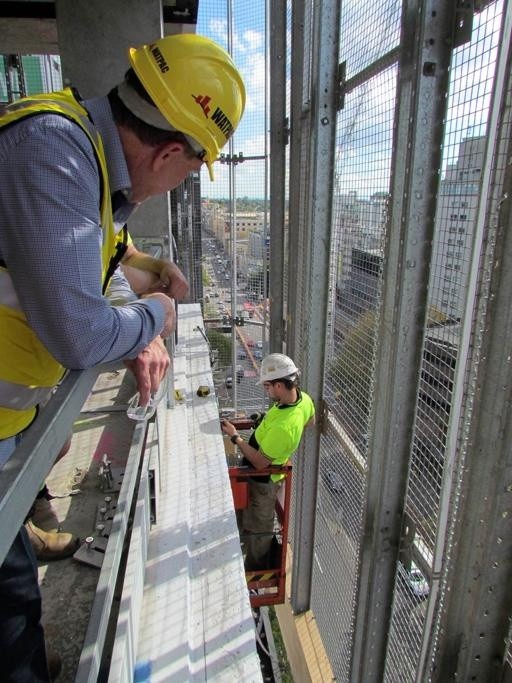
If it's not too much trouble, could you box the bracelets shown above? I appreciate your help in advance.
[230,434,242,444]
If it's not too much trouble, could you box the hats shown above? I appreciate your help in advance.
[254,352,299,386]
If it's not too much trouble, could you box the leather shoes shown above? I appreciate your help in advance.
[22,517,81,563]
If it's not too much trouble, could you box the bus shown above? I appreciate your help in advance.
[242,302,256,318]
[237,308,250,321]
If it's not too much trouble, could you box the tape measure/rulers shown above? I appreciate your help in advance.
[197,385,210,396]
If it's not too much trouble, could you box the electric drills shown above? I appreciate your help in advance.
[220,412,266,430]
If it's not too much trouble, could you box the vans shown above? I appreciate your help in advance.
[225,376,234,388]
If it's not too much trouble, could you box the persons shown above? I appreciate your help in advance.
[220,352,316,572]
[24,228,189,563]
[0,31,247,683]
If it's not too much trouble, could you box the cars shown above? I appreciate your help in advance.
[237,350,247,359]
[253,349,262,360]
[246,337,255,347]
[397,556,430,596]
[203,240,240,321]
[325,468,345,493]
[256,340,263,348]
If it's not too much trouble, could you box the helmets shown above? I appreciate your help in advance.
[118,30,247,182]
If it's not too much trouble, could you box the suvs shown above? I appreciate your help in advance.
[236,363,245,377]
